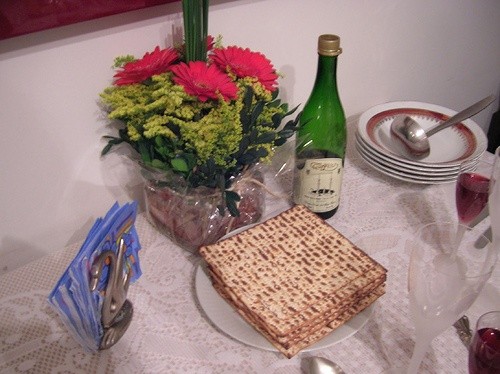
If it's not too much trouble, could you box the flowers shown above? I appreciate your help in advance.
[97,0,305,216]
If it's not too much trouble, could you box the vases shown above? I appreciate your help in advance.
[139,169,267,258]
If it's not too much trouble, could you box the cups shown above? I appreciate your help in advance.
[468,311,500,374]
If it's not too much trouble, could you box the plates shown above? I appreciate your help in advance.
[354,100,487,184]
[195,221,377,351]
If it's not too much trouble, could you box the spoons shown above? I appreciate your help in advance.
[300,356,346,374]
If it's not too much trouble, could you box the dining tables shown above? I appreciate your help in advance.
[0,120,500,373]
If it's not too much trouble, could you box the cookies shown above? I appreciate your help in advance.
[199,205,388,358]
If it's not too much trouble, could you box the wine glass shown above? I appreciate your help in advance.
[435,159,493,272]
[457,148,500,318]
[408,222,498,374]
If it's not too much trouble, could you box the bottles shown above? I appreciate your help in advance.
[292,33,347,220]
